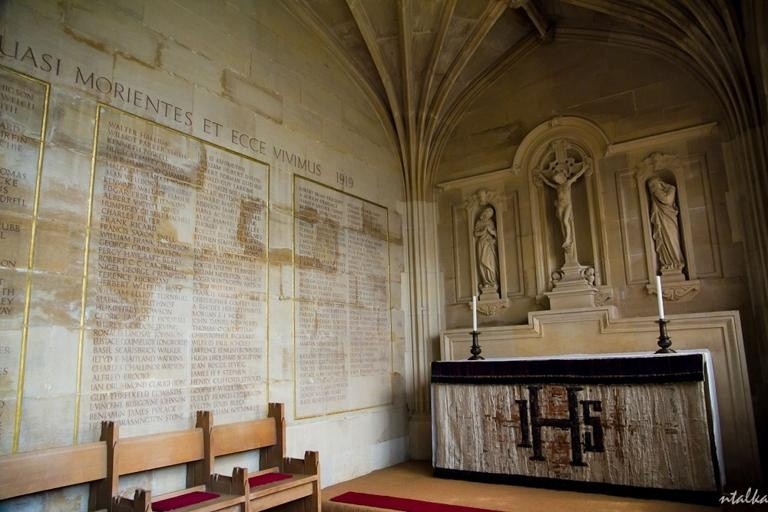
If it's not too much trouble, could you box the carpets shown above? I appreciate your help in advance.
[328,492,499,511]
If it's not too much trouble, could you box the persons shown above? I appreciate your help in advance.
[473,206,499,287]
[537,161,589,248]
[648,179,685,268]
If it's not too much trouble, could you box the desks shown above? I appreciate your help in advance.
[427,346,726,505]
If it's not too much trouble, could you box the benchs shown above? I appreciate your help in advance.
[0,403,320,512]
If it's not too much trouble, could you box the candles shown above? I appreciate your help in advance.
[472,296,477,330]
[654,275,665,319]
